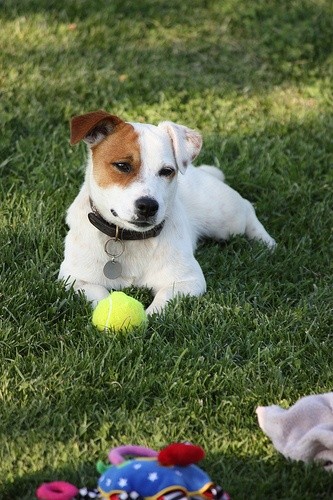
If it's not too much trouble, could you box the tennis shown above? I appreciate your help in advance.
[91,290,146,335]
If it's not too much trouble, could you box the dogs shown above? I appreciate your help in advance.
[56,112,277,317]
